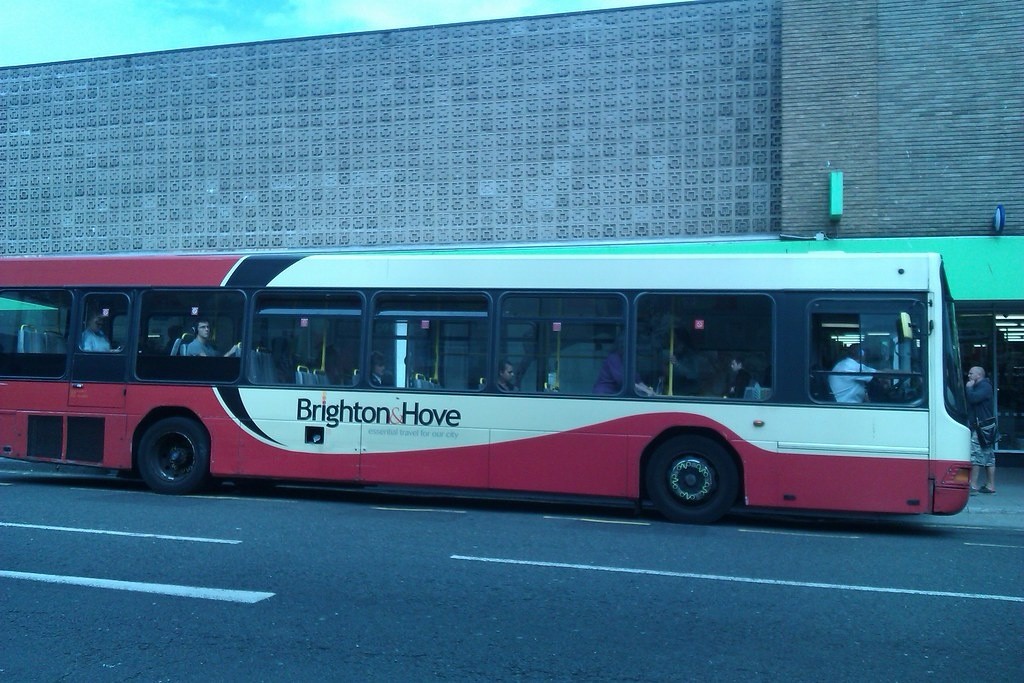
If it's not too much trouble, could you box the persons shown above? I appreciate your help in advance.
[592,332,657,396]
[731,357,754,399]
[498,361,519,391]
[187,317,237,357]
[80,313,110,352]
[657,341,688,396]
[966,366,996,495]
[370,350,389,387]
[903,362,922,401]
[828,343,883,403]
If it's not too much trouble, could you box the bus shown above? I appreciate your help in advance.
[0,245,977,523]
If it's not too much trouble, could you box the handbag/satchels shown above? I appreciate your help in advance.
[975,416,1003,449]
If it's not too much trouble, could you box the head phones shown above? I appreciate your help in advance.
[192,316,213,333]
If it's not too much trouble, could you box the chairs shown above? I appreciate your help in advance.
[3,315,888,403]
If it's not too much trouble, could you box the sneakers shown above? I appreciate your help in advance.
[969,487,978,496]
[979,484,996,495]
